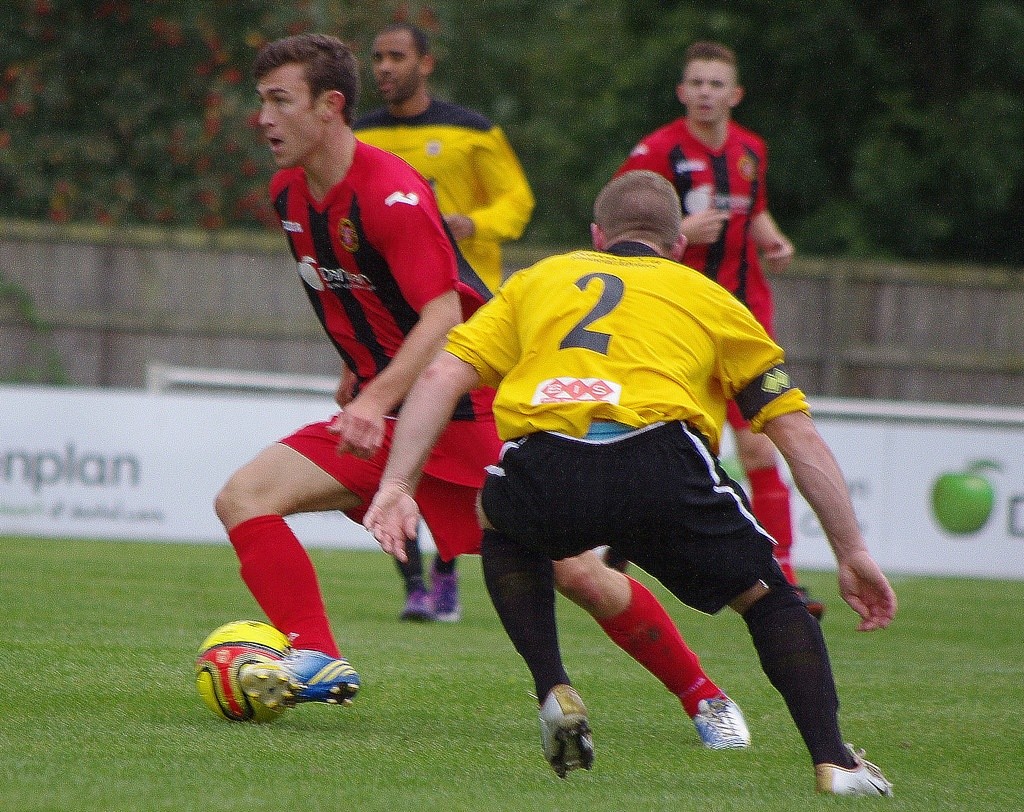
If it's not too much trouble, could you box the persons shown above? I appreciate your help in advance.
[336,21,533,623]
[361,167,897,800]
[609,42,827,620]
[214,33,752,752]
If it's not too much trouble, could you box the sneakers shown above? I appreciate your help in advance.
[402,589,433,621]
[240,648,361,706]
[692,691,751,753]
[539,685,594,778]
[432,558,457,623]
[817,741,895,797]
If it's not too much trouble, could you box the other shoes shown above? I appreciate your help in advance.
[601,547,629,573]
[795,585,825,621]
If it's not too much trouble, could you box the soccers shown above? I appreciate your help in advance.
[193,618,296,726]
